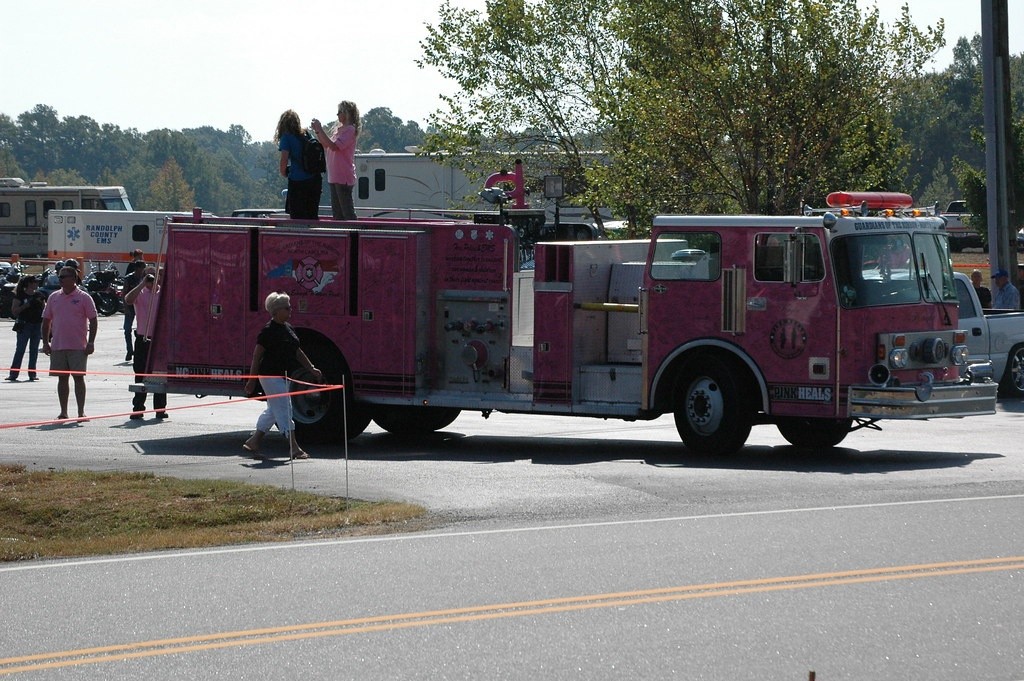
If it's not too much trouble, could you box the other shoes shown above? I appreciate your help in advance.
[30,377,39,381]
[56,416,67,419]
[129,414,143,419]
[156,412,168,419]
[5,377,16,381]
[126,351,134,361]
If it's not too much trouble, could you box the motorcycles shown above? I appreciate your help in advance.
[0,262,125,321]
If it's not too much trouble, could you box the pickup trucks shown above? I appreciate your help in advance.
[940,200,989,253]
[863,270,1023,398]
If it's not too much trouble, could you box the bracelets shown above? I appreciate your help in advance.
[87,342,94,344]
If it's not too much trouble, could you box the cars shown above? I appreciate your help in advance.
[0,262,12,275]
[1016,229,1024,255]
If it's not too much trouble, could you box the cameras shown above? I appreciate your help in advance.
[311,121,315,127]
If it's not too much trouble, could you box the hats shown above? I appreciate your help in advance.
[991,270,1009,279]
[23,276,42,285]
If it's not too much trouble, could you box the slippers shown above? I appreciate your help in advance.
[292,451,307,459]
[243,445,266,458]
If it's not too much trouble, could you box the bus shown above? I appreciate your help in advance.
[0,178,134,258]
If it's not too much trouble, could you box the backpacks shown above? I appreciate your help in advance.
[290,130,327,174]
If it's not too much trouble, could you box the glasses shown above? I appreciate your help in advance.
[280,306,291,311]
[58,274,73,279]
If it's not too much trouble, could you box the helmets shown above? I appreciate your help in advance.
[55,261,64,269]
[66,259,80,268]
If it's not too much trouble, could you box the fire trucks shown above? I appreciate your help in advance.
[129,159,998,457]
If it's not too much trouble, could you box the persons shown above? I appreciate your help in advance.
[4,275,45,380]
[310,100,362,220]
[40,266,98,422]
[45,258,83,302]
[125,249,144,276]
[244,292,322,459]
[273,109,323,220]
[124,266,169,419]
[970,269,992,309]
[123,260,147,361]
[990,269,1021,309]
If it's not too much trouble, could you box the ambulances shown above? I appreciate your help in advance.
[47,209,218,278]
